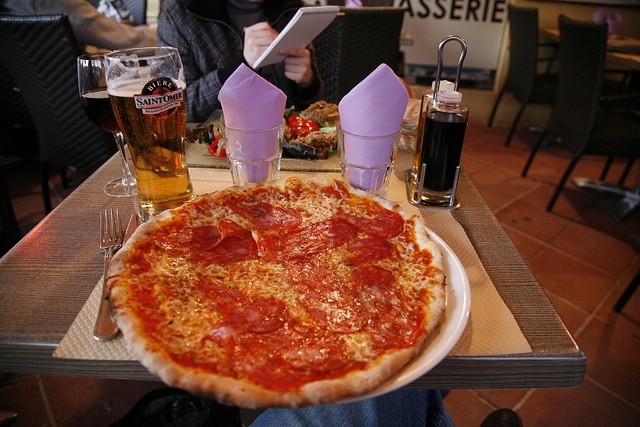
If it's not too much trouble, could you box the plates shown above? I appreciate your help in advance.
[329,201,473,405]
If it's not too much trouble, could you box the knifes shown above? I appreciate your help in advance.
[122,212,137,246]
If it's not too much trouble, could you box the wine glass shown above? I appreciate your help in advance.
[77,51,142,197]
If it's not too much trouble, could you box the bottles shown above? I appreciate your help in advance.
[398,123,418,154]
[411,79,457,175]
[416,89,470,192]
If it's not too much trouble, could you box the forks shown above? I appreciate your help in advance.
[92,207,124,341]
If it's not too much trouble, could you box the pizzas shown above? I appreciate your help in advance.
[103,174,447,411]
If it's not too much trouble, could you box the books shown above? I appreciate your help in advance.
[252,6,340,69]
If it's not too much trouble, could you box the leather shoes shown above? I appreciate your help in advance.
[480,408,523,426]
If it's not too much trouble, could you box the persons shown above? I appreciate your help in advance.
[0,0,159,56]
[249,386,523,427]
[155,0,324,122]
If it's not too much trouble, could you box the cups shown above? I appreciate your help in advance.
[335,122,403,200]
[102,45,199,223]
[219,114,286,186]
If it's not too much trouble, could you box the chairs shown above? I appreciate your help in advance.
[312,4,408,105]
[1,13,100,214]
[484,5,561,148]
[522,12,639,213]
